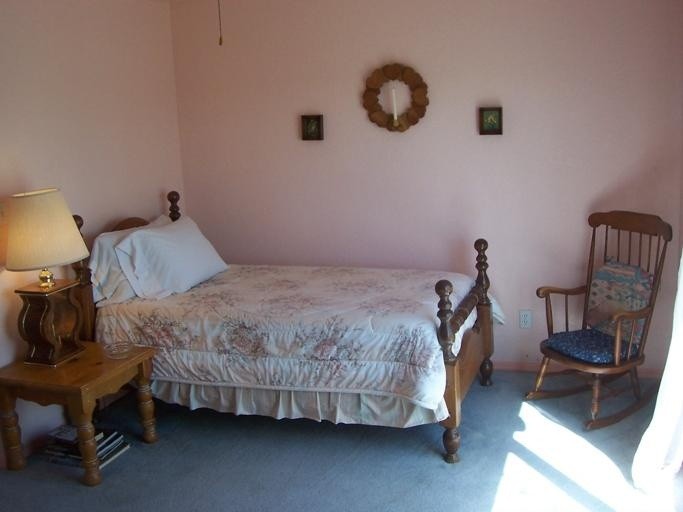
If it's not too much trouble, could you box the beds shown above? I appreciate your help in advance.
[72,190,497,466]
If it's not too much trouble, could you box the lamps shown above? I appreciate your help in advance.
[1,185,91,370]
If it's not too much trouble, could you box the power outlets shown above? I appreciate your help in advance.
[519,310,532,329]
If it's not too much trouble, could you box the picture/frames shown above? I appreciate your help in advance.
[478,107,503,136]
[301,114,324,141]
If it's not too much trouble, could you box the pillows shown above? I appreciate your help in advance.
[87,210,230,309]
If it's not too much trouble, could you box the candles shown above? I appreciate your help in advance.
[391,88,397,120]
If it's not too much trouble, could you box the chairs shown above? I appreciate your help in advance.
[523,208,674,433]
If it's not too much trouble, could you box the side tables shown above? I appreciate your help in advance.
[0,341,162,487]
